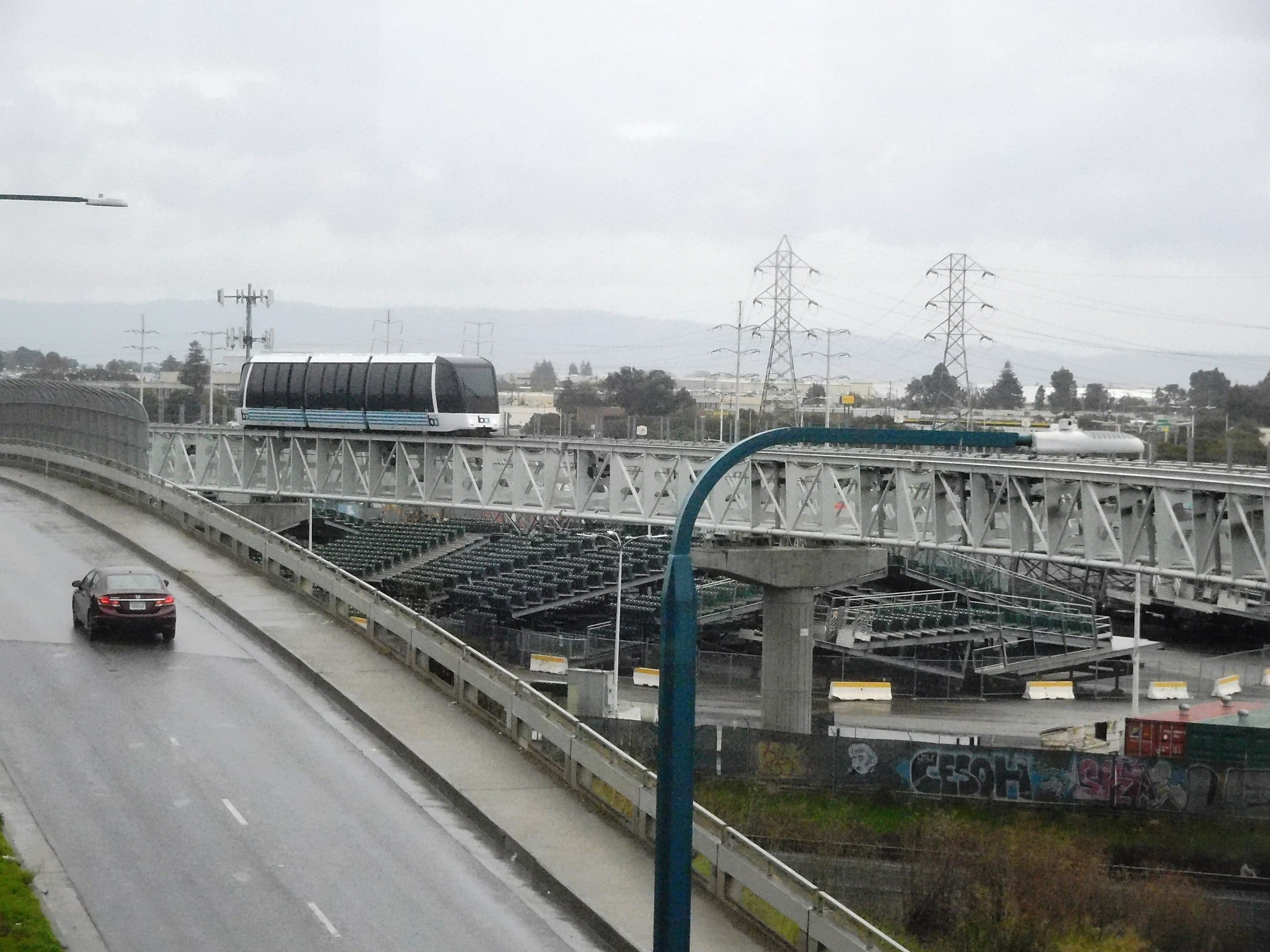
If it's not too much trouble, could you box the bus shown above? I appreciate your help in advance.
[234,351,501,438]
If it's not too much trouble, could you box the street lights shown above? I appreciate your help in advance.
[576,530,669,713]
[650,415,1147,952]
[121,313,160,404]
[193,330,228,426]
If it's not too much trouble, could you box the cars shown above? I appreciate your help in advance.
[72,565,177,641]
